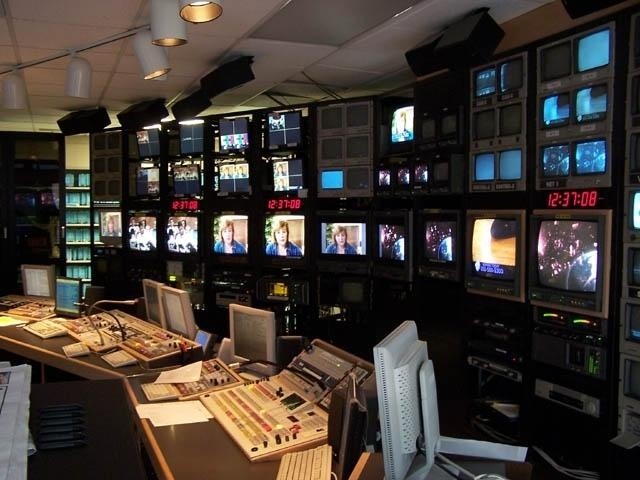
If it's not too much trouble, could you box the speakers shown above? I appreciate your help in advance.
[200,62,255,99]
[404,29,449,76]
[116,97,170,131]
[171,90,212,120]
[560,0,625,20]
[218,338,232,364]
[435,11,506,73]
[194,329,217,359]
[329,387,380,463]
[56,107,111,136]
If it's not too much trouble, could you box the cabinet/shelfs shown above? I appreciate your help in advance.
[66,168,101,284]
[12,134,63,280]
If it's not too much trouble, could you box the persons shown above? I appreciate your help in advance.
[392,112,413,142]
[129,218,157,251]
[103,215,119,237]
[273,163,288,176]
[167,218,197,254]
[220,167,234,179]
[214,220,246,254]
[235,167,247,178]
[324,226,358,254]
[266,220,303,256]
[274,179,287,192]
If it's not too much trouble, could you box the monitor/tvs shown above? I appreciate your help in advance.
[372,319,441,480]
[574,28,610,73]
[165,154,206,200]
[163,212,205,261]
[142,278,166,330]
[98,208,122,245]
[498,148,522,180]
[218,117,249,154]
[135,128,161,158]
[473,151,495,182]
[538,142,570,177]
[315,211,370,263]
[180,124,204,153]
[126,211,158,253]
[571,136,607,177]
[320,138,342,160]
[372,216,408,266]
[414,164,428,184]
[473,107,495,139]
[498,56,524,93]
[539,39,571,83]
[464,207,525,301]
[473,63,496,97]
[129,159,161,202]
[230,303,275,381]
[389,106,414,144]
[321,106,342,129]
[209,209,251,262]
[347,103,368,128]
[271,157,305,192]
[525,206,613,316]
[422,120,435,139]
[441,114,457,135]
[55,277,82,317]
[346,135,370,159]
[258,211,311,265]
[377,168,391,186]
[572,83,608,125]
[160,285,199,340]
[396,167,411,186]
[320,170,344,190]
[214,157,252,199]
[539,92,571,131]
[347,166,370,189]
[497,102,523,137]
[21,264,56,298]
[418,209,459,281]
[433,162,449,182]
[628,192,640,231]
[266,110,303,150]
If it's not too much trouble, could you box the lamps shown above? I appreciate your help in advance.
[2,65,26,109]
[65,49,93,100]
[149,0,188,47]
[132,25,172,80]
[179,0,223,24]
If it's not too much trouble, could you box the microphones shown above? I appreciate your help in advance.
[74,303,125,341]
[88,300,134,352]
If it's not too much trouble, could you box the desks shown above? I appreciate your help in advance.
[121,363,533,480]
[0,270,203,379]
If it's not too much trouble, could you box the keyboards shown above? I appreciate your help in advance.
[62,341,91,357]
[140,382,177,401]
[275,445,332,480]
[22,320,69,338]
[100,349,138,368]
